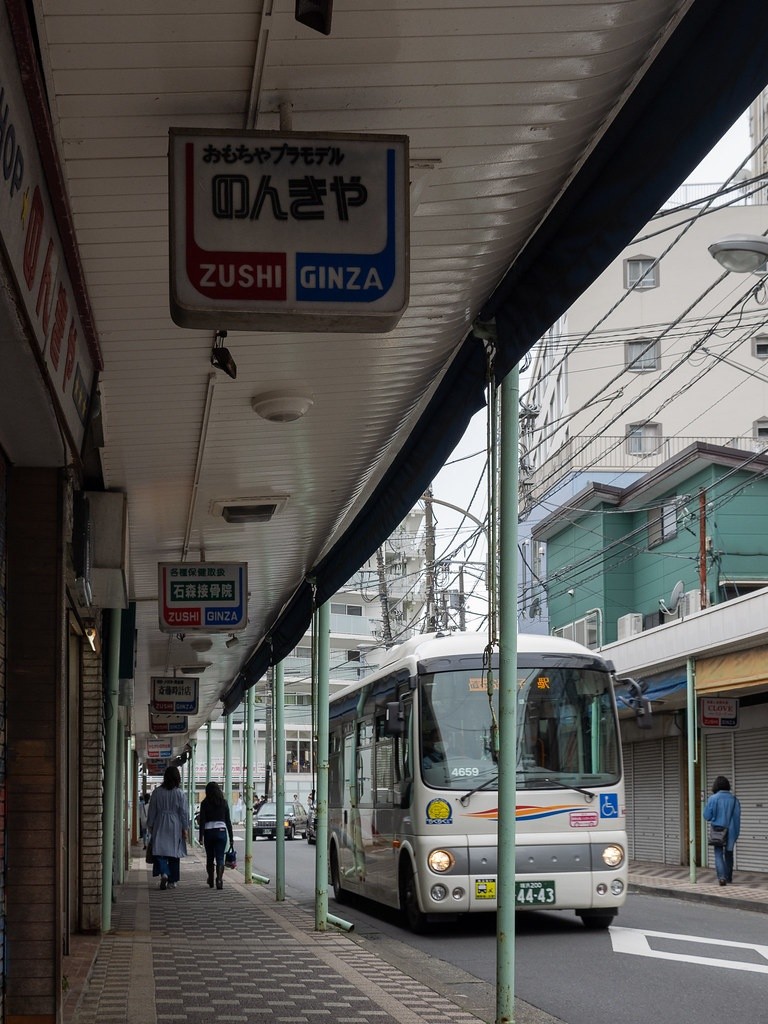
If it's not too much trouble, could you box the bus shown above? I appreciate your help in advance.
[314,629,653,935]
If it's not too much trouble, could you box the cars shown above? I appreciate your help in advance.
[253,801,308,841]
[306,800,317,845]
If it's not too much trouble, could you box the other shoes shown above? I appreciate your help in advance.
[143,846,147,851]
[168,883,177,889]
[719,878,732,887]
[160,874,168,890]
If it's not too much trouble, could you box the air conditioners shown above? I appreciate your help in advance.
[680,589,710,616]
[617,612,643,641]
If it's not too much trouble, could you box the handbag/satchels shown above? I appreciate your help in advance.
[225,844,237,869]
[708,796,737,846]
[146,837,153,864]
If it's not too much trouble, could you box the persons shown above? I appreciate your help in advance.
[147,767,190,891]
[703,776,741,886]
[273,755,304,772]
[138,793,150,850]
[422,727,454,770]
[236,794,272,814]
[199,781,233,889]
[293,794,299,803]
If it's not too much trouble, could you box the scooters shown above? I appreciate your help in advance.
[243,802,259,829]
[194,806,200,830]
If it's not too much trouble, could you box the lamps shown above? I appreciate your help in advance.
[251,391,314,422]
[192,640,213,651]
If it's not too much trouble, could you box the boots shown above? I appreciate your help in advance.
[206,863,214,888]
[216,864,225,889]
